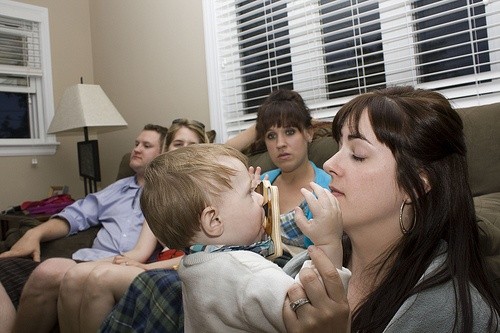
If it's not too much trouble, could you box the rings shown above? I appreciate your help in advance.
[289,298,310,312]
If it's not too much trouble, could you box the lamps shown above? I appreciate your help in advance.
[46,74,128,195]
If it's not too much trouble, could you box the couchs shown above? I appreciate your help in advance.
[0,101,500,272]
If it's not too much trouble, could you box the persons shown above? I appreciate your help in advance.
[99,90,331,333]
[0,124,168,333]
[283,85,500,333]
[140,144,352,333]
[57,119,321,333]
